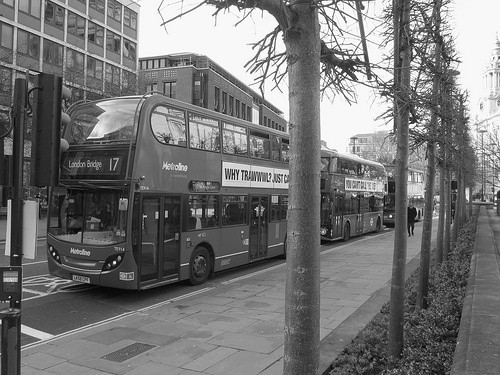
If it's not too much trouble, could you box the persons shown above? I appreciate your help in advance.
[407,202,417,237]
[101,202,112,228]
[207,215,217,227]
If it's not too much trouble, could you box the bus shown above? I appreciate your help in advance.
[385,165,425,228]
[321,150,388,241]
[45,91,288,291]
